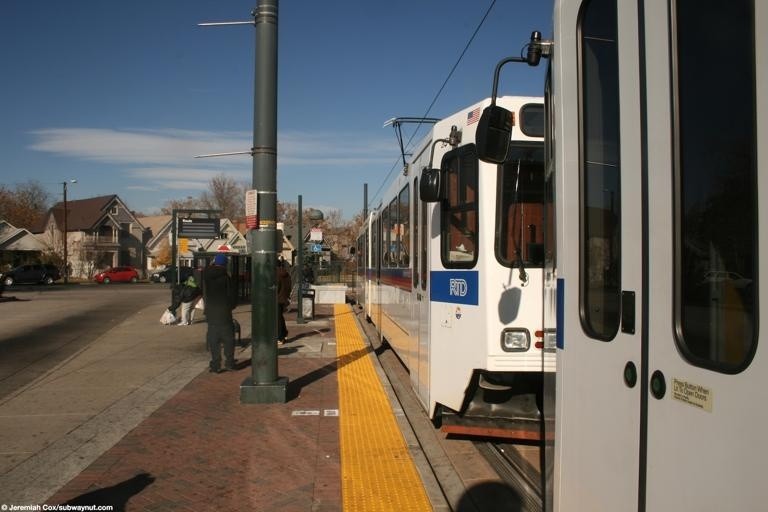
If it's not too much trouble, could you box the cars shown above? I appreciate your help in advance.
[0,264,62,287]
[150,265,195,283]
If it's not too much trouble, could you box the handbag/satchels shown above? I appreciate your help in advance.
[160,309,176,324]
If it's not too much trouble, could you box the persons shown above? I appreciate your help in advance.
[275,262,291,345]
[167,282,201,326]
[198,252,238,373]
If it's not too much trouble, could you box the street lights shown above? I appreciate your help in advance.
[61,179,81,277]
[95,266,140,284]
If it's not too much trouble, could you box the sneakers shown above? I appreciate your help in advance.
[209,362,236,372]
[177,320,194,326]
[278,338,287,344]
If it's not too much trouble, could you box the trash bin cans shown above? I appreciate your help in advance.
[301,289,314,320]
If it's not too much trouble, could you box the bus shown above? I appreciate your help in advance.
[348,94,547,444]
[472,0,766,510]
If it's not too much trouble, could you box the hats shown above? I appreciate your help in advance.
[215,254,228,264]
